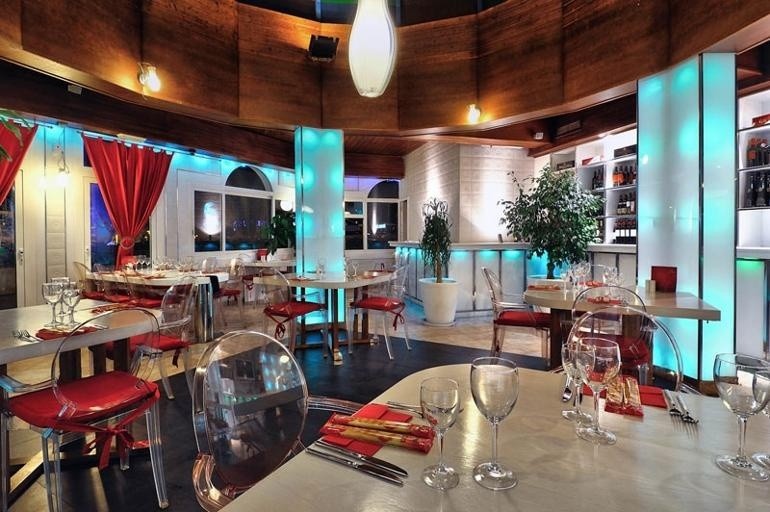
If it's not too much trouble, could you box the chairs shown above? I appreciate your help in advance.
[483,268,722,392]
[253,257,412,366]
[1,256,307,512]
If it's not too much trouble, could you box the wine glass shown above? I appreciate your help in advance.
[470,354,525,491]
[40,277,83,330]
[561,337,620,446]
[558,263,633,305]
[311,256,380,281]
[715,354,770,480]
[419,376,462,488]
[116,253,217,278]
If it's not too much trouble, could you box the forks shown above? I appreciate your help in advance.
[663,387,700,428]
[11,329,41,348]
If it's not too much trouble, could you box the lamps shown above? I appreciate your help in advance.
[307,33,341,64]
[137,60,162,93]
[347,0,398,99]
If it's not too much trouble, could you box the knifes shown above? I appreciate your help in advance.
[305,440,408,486]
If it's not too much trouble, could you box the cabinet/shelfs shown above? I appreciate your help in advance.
[606,151,638,243]
[576,158,606,243]
[735,119,770,248]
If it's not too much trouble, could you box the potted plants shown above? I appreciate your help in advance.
[497,163,606,315]
[417,195,459,328]
[261,210,296,262]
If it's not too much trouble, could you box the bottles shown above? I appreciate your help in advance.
[585,167,604,240]
[744,137,770,206]
[614,163,637,243]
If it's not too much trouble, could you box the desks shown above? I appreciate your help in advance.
[219,363,770,512]
